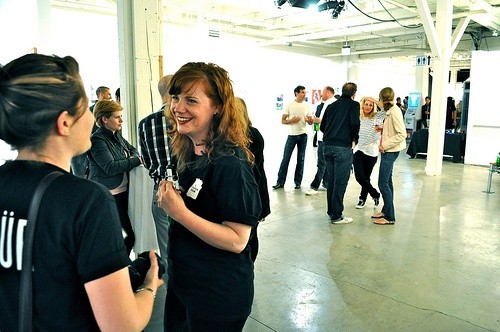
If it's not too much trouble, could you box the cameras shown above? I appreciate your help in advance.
[130,251,166,292]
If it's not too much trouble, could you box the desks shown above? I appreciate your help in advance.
[406,129,466,163]
[483,162,500,193]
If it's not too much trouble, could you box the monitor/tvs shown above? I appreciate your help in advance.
[408,92,421,108]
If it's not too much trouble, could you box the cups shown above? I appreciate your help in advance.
[129,251,166,293]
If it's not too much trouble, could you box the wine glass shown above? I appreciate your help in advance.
[374,119,379,132]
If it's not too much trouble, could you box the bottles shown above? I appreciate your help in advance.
[314,116,319,131]
[167,175,183,225]
[451,122,455,133]
[496,153,500,166]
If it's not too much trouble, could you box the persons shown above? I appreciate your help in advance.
[0,53,165,332]
[371,87,407,225]
[313,95,325,147]
[352,96,387,209]
[86,99,143,258]
[138,74,180,286]
[305,86,338,195]
[396,96,408,118]
[115,88,120,103]
[445,96,457,128]
[156,61,261,332]
[320,82,361,224]
[71,153,88,178]
[272,85,314,190]
[89,86,112,133]
[236,97,271,263]
[421,96,431,128]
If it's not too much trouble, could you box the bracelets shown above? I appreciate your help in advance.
[135,288,156,301]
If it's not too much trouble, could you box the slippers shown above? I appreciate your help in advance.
[373,218,395,225]
[371,213,385,218]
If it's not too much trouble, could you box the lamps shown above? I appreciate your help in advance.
[274,0,348,19]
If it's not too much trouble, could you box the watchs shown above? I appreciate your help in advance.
[288,120,290,124]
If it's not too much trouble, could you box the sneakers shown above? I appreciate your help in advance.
[273,184,284,189]
[374,194,380,208]
[333,217,353,224]
[295,184,301,189]
[355,200,365,208]
[317,185,327,190]
[305,189,318,195]
